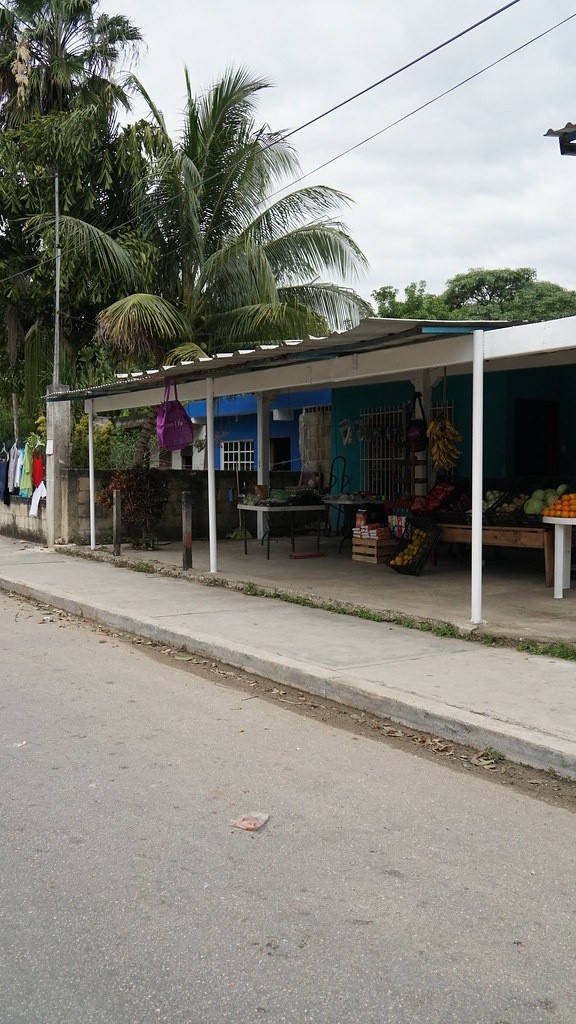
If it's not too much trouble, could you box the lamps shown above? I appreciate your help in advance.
[191,416,207,425]
[273,389,294,421]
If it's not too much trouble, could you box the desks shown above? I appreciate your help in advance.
[431,523,553,588]
[322,500,386,555]
[541,516,576,599]
[237,504,325,560]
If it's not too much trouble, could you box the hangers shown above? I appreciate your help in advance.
[32,437,45,452]
[0,442,9,461]
[26,441,29,445]
[12,439,18,450]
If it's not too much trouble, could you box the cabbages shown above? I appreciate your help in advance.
[524,483,568,514]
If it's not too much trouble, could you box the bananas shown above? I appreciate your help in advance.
[426,416,463,471]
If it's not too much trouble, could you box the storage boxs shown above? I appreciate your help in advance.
[352,478,576,576]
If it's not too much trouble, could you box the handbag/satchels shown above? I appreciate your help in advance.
[157,381,194,449]
[406,392,429,452]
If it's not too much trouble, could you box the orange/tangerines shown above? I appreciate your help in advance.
[542,493,576,518]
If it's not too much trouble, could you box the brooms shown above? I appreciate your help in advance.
[231,461,253,540]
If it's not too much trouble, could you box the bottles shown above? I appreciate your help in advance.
[391,507,410,518]
[355,508,367,527]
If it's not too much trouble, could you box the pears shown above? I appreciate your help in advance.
[394,528,427,565]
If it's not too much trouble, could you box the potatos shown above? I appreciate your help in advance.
[495,494,528,517]
